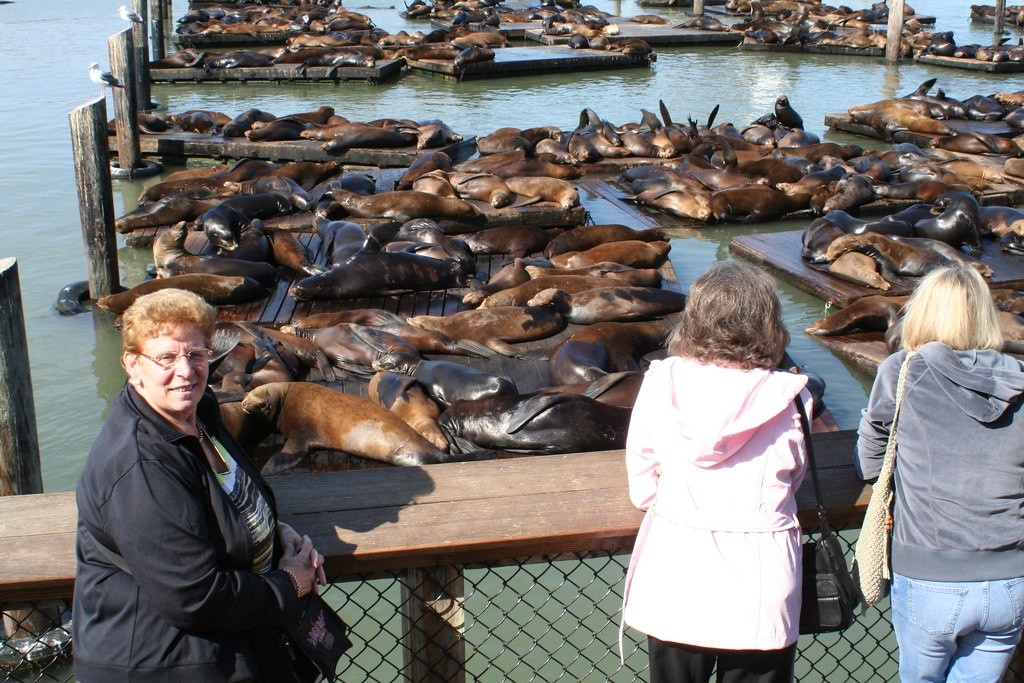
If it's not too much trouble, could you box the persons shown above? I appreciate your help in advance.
[622,260,815,683]
[853,267,1024,683]
[72,287,327,683]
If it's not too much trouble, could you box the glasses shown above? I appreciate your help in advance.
[138,348,210,368]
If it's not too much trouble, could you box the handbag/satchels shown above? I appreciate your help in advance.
[239,584,352,683]
[852,442,915,605]
[784,510,859,636]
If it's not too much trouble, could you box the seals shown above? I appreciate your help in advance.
[96,0,1024,476]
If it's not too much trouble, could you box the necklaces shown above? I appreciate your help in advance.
[195,421,203,443]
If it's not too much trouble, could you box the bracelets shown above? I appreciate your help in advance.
[280,568,300,598]
